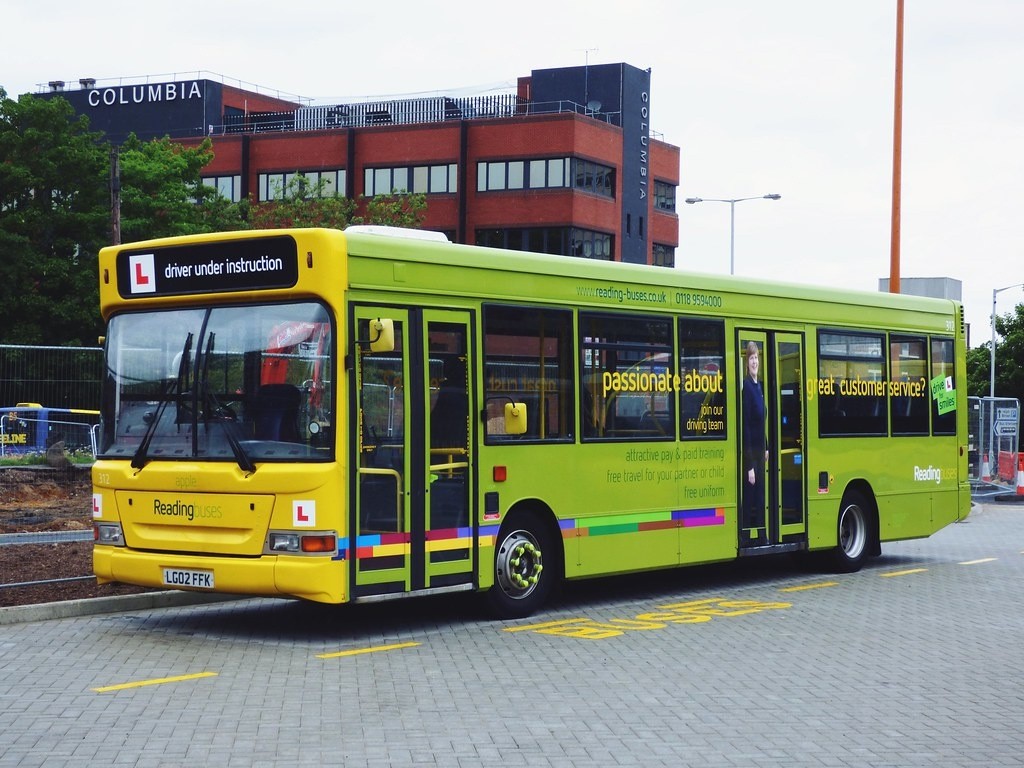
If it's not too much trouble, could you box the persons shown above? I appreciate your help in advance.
[741,341,768,548]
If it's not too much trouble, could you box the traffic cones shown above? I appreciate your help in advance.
[981,454,991,482]
[1015,463,1024,496]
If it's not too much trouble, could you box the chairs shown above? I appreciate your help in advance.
[779,376,950,434]
[243,382,301,443]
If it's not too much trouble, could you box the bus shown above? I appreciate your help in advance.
[0,403,101,456]
[88,227,973,620]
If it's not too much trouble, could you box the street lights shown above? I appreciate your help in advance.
[681,193,781,277]
